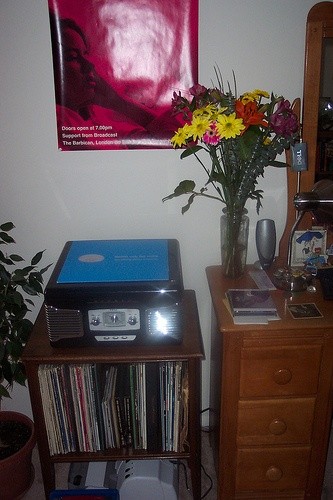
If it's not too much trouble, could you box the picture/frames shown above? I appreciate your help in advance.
[288,229,327,266]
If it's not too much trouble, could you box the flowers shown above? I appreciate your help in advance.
[163,62,301,213]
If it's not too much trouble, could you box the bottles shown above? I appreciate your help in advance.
[220,207,249,278]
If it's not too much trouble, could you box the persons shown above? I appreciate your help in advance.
[50,18,184,150]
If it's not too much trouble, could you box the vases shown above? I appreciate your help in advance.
[220,214,248,277]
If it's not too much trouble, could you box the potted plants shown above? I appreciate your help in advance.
[0,221,52,500]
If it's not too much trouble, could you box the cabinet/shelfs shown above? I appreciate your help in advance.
[205,264,332,500]
[20,292,207,500]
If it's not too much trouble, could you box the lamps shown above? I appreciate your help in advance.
[272,180,333,292]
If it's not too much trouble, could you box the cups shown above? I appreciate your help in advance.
[256,219,276,269]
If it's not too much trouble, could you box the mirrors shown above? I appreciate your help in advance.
[303,2,333,185]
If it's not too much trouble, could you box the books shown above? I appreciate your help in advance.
[222,288,282,324]
[36,362,189,456]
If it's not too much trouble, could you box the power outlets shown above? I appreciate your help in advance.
[289,142,309,172]
